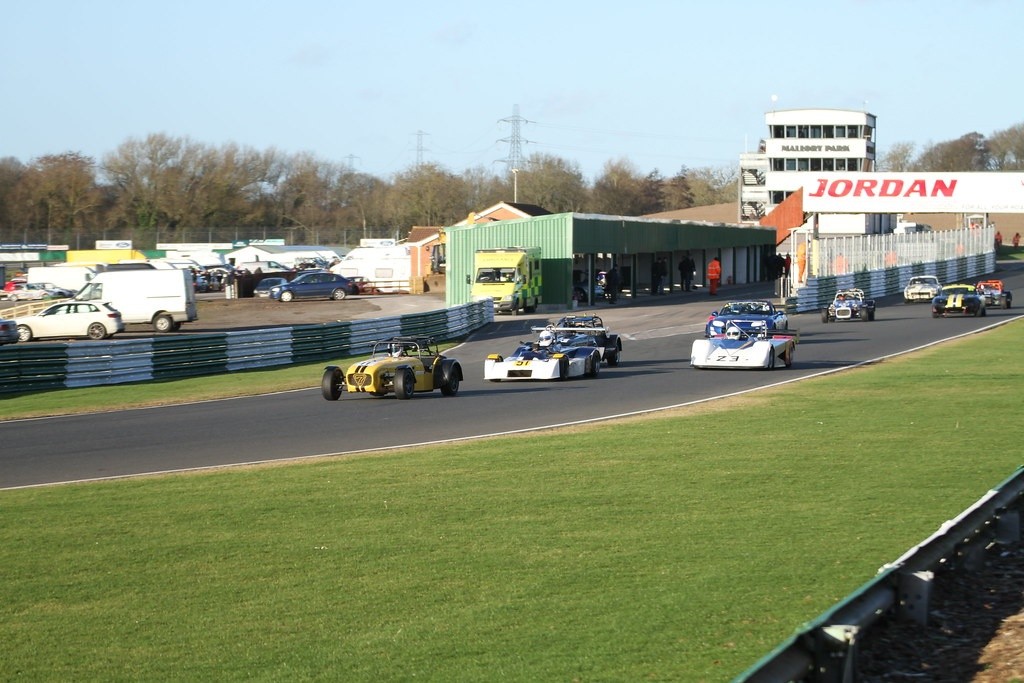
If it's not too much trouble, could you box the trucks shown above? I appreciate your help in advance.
[328,243,411,293]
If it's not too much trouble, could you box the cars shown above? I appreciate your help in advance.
[268,272,355,302]
[253,277,288,298]
[6,299,126,344]
[1,272,74,303]
[0,317,22,346]
[116,257,333,293]
[572,268,606,302]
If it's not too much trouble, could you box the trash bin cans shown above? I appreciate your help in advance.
[211,276,222,291]
[199,276,210,293]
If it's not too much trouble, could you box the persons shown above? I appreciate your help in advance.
[707,257,721,295]
[835,254,846,274]
[678,254,696,291]
[532,330,553,351]
[605,264,619,304]
[573,323,585,333]
[777,253,791,278]
[796,241,806,282]
[996,231,1002,244]
[885,249,897,267]
[391,344,404,358]
[1013,232,1020,247]
[650,257,668,296]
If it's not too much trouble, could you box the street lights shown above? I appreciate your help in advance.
[511,168,519,202]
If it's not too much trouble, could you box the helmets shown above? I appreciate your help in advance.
[730,305,741,314]
[726,327,741,340]
[539,331,553,347]
[391,344,404,357]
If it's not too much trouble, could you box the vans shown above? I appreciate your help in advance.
[26,264,102,292]
[57,267,200,333]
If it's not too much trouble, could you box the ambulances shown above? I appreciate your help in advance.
[465,245,542,316]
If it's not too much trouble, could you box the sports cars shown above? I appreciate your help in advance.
[931,282,987,318]
[903,275,942,305]
[551,313,623,355]
[705,299,789,340]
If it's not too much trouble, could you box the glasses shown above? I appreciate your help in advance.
[539,336,551,342]
[727,331,739,336]
[391,346,400,353]
